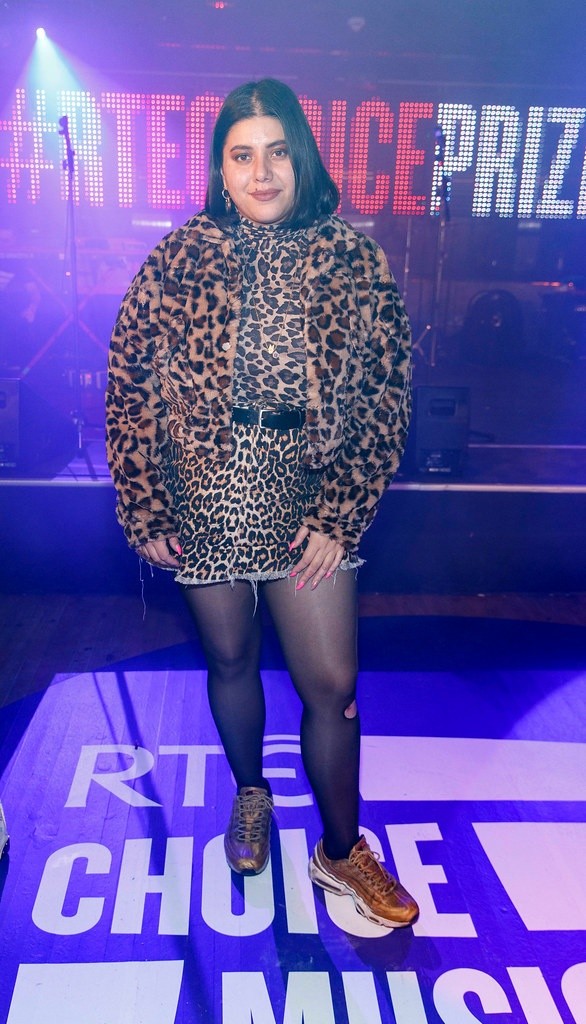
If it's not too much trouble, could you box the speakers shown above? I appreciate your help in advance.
[405,386,471,475]
[0,377,58,473]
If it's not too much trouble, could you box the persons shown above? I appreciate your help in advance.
[103,78,421,929]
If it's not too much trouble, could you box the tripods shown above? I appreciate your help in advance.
[413,160,458,366]
[64,170,104,458]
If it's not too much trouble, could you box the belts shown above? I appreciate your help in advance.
[232,407,308,431]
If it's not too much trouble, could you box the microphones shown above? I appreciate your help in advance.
[60,116,73,172]
[433,128,445,162]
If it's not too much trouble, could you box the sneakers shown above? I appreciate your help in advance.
[308,834,420,928]
[223,777,280,879]
